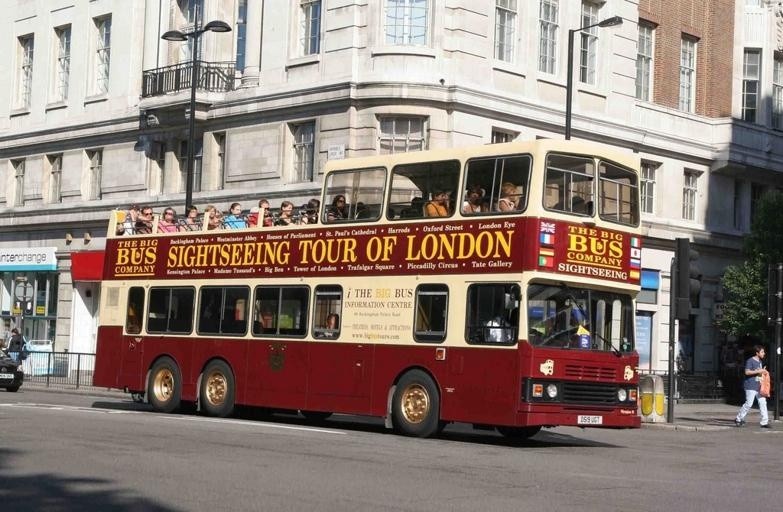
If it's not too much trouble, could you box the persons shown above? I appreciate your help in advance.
[328,195,349,221]
[157,205,203,234]
[116,204,154,235]
[388,208,395,219]
[735,344,771,428]
[572,196,593,216]
[317,313,339,339]
[357,202,370,219]
[462,183,526,214]
[274,201,297,226]
[295,199,320,225]
[2,327,27,365]
[426,189,457,217]
[400,197,425,217]
[205,199,270,230]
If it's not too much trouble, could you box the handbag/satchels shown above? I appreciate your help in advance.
[760,371,771,397]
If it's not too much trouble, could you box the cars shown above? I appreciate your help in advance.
[0,348,25,391]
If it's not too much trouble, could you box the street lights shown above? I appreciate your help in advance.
[160,6,232,218]
[566,15,623,141]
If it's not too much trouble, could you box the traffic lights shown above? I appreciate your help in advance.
[676,238,701,318]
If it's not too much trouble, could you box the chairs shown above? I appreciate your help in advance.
[174,206,423,229]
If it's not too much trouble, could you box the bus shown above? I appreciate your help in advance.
[92,136,642,441]
